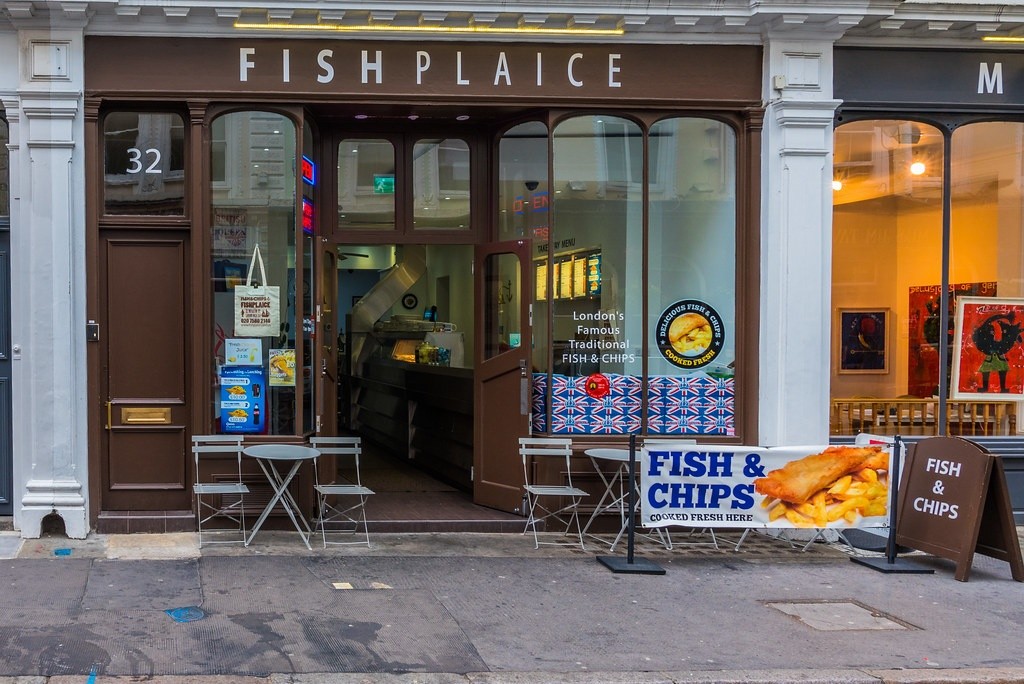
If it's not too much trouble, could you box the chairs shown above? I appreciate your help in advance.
[829,394,935,436]
[191,435,249,549]
[642,439,722,552]
[309,436,375,549]
[518,437,591,550]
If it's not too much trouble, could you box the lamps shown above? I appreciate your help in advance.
[568,180,588,191]
[892,125,922,144]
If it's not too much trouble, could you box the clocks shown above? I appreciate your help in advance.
[403,294,418,309]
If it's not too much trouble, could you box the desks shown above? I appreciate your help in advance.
[578,448,674,550]
[850,407,995,436]
[243,444,322,552]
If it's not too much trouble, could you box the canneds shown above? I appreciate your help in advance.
[252,384,260,398]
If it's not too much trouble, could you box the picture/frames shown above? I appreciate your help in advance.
[951,295,1024,398]
[835,307,891,375]
[352,296,363,307]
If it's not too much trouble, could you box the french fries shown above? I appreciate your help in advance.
[760,468,888,527]
[673,327,712,353]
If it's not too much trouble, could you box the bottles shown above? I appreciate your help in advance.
[416,340,450,369]
[253,403,260,425]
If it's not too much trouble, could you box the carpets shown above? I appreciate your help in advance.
[335,444,458,493]
